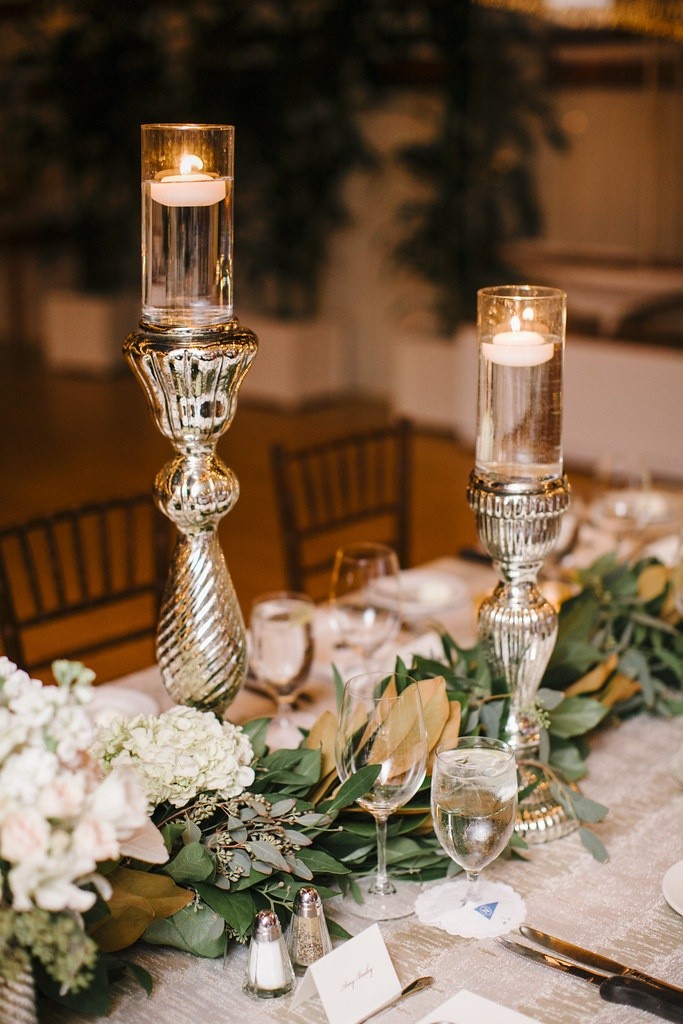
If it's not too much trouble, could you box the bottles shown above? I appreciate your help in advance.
[243,908,297,1001]
[288,888,332,976]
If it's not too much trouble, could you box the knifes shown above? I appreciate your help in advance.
[519,925,683,999]
[497,935,683,1024]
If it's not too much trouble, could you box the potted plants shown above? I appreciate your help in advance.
[0,0,561,421]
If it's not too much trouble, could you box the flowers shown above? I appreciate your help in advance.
[0,516,672,993]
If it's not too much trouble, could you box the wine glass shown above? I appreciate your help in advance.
[432,736,517,917]
[331,543,402,675]
[333,673,427,920]
[249,591,317,753]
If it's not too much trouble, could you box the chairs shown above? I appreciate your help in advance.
[272,418,416,598]
[0,485,172,695]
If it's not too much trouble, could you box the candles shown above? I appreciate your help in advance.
[146,154,226,206]
[486,313,554,370]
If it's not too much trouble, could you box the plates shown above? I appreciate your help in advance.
[662,859,683,916]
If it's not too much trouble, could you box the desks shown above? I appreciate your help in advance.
[2,555,679,1023]
